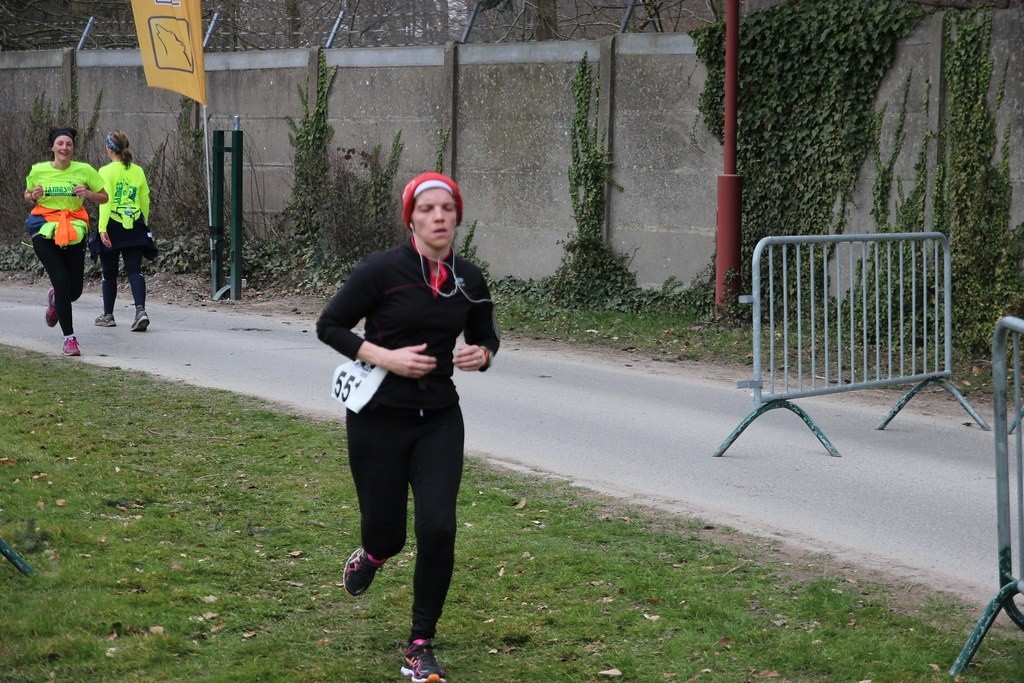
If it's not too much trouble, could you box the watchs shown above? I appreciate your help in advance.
[481,344,494,367]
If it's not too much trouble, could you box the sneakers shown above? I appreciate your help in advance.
[46,289,58,327]
[94,313,116,327]
[62,335,81,356]
[131,311,150,331]
[400,638,447,683]
[343,547,387,597]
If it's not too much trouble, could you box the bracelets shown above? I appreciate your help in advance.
[29,194,37,202]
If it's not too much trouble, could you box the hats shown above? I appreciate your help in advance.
[401,172,464,230]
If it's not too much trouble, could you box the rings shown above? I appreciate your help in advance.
[78,189,80,192]
[473,359,478,365]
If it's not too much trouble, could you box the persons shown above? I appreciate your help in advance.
[316,171,498,683]
[97,129,149,333]
[25,126,109,356]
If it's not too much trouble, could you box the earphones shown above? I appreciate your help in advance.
[410,223,414,231]
